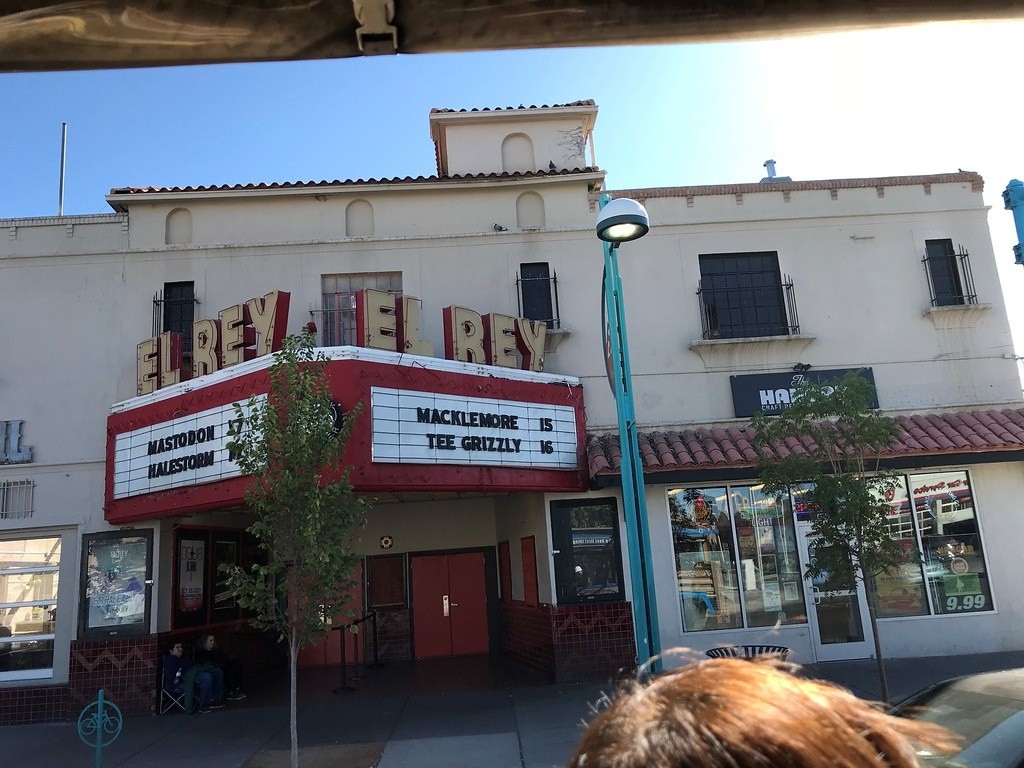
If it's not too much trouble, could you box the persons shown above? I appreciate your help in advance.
[568,647,966,768]
[162,631,251,713]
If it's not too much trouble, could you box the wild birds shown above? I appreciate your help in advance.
[492,222,508,232]
[548,159,557,170]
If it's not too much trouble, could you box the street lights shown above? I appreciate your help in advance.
[596,191,666,683]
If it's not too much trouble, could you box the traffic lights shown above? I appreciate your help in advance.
[694,494,704,514]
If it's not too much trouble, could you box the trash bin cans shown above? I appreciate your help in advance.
[705,644,789,663]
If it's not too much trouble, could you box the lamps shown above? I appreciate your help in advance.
[792,363,812,371]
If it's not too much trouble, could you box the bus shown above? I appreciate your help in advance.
[569,523,723,633]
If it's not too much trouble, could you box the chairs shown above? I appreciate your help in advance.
[159,646,215,714]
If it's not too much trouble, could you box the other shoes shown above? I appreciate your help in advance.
[199,705,213,714]
[209,700,226,709]
